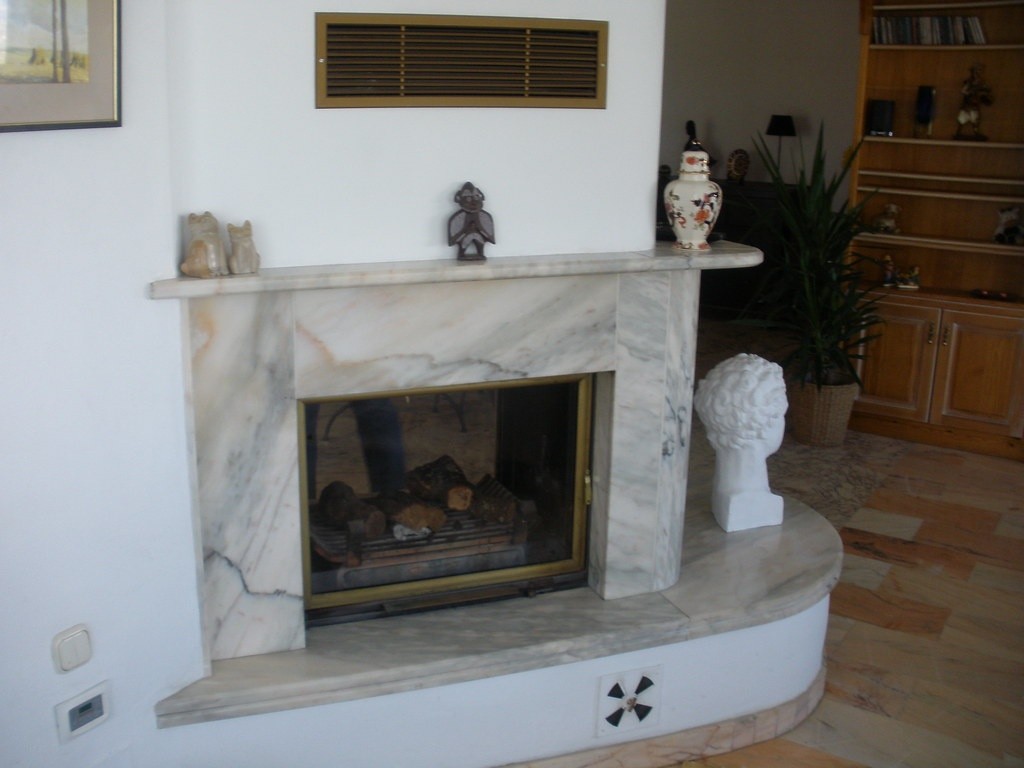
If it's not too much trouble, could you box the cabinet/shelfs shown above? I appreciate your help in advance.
[831,0,1024,464]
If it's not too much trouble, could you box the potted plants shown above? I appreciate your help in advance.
[701,120,903,447]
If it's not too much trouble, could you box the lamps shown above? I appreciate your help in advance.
[767,115,798,187]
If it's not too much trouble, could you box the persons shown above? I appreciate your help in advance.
[955,66,985,136]
[693,352,789,533]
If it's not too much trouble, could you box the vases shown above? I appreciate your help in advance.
[663,138,722,251]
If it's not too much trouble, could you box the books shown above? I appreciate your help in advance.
[872,16,986,45]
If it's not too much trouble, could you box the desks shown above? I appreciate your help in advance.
[657,176,812,324]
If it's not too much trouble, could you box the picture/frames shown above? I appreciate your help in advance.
[0,0,123,132]
